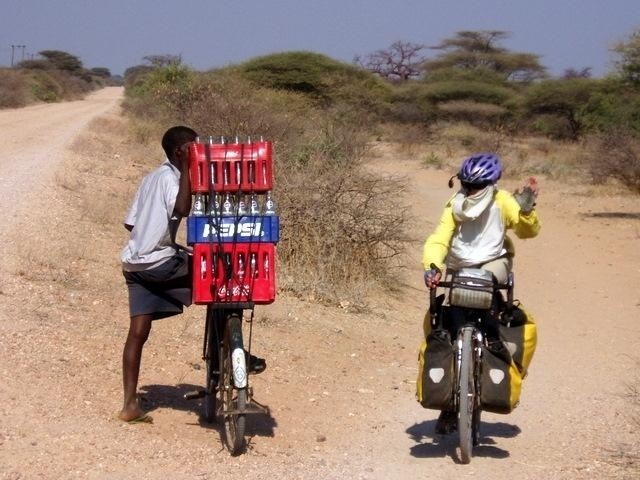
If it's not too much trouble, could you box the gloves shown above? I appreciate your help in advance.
[423,262,443,288]
[513,186,538,213]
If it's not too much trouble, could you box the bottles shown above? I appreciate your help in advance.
[193,136,274,279]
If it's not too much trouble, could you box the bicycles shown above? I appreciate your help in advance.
[184,302,270,455]
[429,263,514,464]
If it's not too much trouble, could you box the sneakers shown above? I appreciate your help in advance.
[244,351,266,375]
[434,413,458,438]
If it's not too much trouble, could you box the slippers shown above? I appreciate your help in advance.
[118,410,154,426]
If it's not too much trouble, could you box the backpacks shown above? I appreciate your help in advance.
[478,339,523,415]
[447,266,498,311]
[422,295,442,339]
[496,297,538,380]
[414,326,458,412]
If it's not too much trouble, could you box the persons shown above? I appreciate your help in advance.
[420,152,539,437]
[117,125,268,426]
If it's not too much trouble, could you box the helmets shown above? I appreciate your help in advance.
[457,153,502,190]
